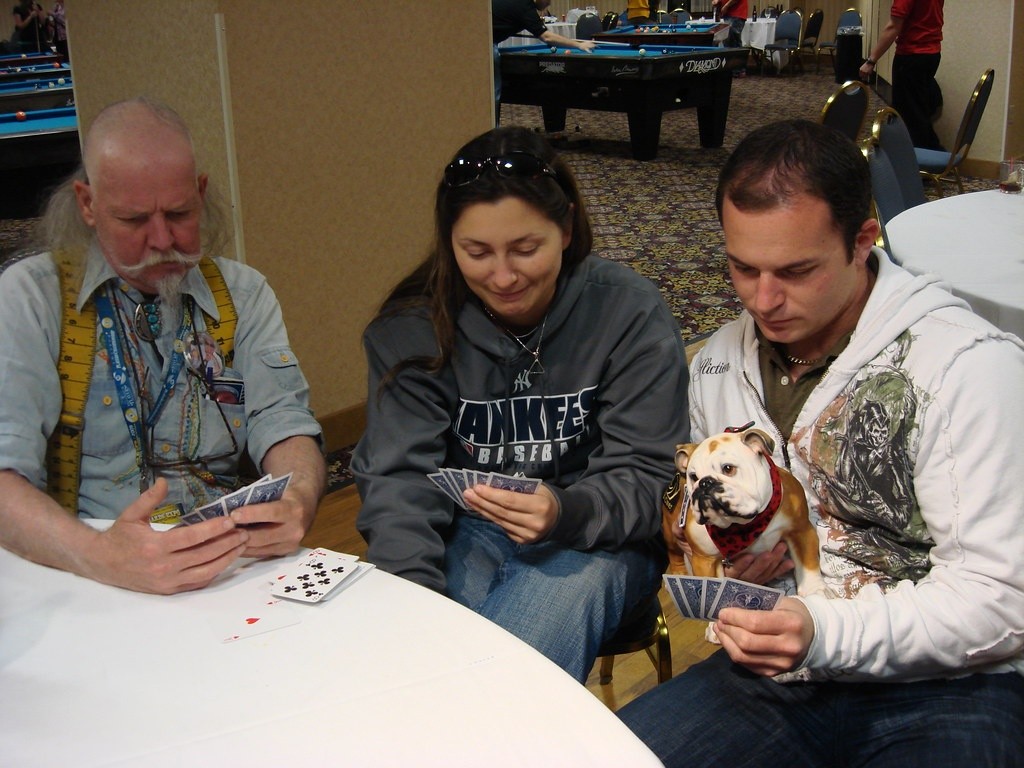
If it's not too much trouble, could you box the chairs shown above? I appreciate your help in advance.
[593,601,674,687]
[818,68,995,267]
[576,6,878,93]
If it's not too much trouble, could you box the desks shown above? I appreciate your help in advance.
[740,16,790,74]
[591,23,731,47]
[0,519,661,768]
[884,188,1024,341]
[0,51,81,219]
[497,40,750,161]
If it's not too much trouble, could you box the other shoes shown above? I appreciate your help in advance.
[733,72,746,78]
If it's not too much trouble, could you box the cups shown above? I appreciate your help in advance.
[562,15,566,22]
[999,161,1024,194]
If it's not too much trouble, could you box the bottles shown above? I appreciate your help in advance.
[713,5,720,22]
[775,4,784,20]
[752,5,757,22]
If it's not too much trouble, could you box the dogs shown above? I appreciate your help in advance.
[660,428,823,643]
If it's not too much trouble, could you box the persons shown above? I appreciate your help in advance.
[858,0,944,179]
[350,128,687,684]
[490,0,600,123]
[619,118,1024,768]
[712,0,747,77]
[13,0,68,60]
[0,94,332,597]
[626,0,659,25]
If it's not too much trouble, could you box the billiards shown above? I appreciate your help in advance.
[58,77,65,86]
[21,54,26,58]
[54,62,60,68]
[48,81,55,88]
[17,111,26,121]
[35,85,42,90]
[635,25,697,34]
[662,48,696,55]
[551,47,557,52]
[639,48,646,56]
[1,67,36,74]
[565,49,570,53]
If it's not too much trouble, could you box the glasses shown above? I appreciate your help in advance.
[141,366,240,467]
[444,151,559,187]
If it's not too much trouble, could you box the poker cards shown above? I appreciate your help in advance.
[178,470,294,526]
[426,468,543,516]
[662,574,786,623]
[196,548,376,643]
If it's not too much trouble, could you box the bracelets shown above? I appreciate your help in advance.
[866,58,876,65]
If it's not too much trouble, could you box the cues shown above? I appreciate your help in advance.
[214,12,247,265]
[509,33,630,47]
[33,1,40,52]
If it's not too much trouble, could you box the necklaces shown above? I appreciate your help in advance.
[787,351,819,366]
[113,282,164,341]
[481,307,549,379]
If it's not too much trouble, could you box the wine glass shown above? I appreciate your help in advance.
[765,8,772,22]
